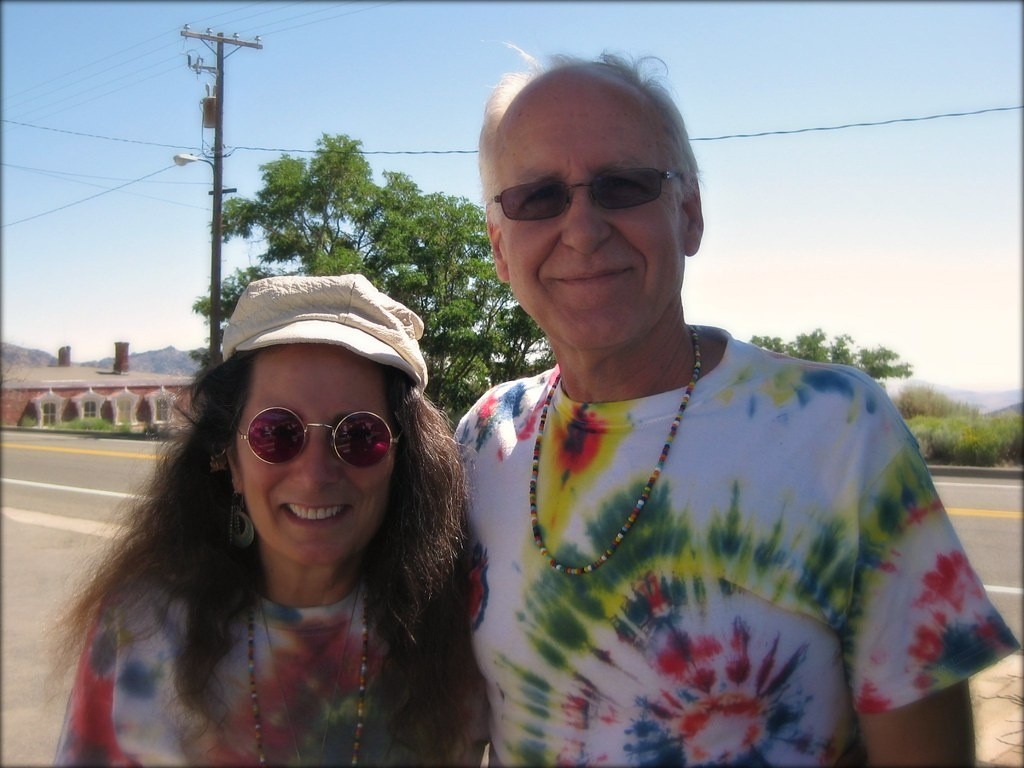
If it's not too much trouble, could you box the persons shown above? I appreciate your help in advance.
[437,62,1022,768]
[40,274,489,767]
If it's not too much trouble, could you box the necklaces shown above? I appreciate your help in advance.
[529,325,704,577]
[245,571,373,768]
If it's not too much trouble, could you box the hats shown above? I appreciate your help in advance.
[221,273,429,397]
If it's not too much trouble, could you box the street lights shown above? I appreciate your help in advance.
[175,153,222,369]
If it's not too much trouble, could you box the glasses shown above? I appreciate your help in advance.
[493,169,684,222]
[237,406,402,468]
